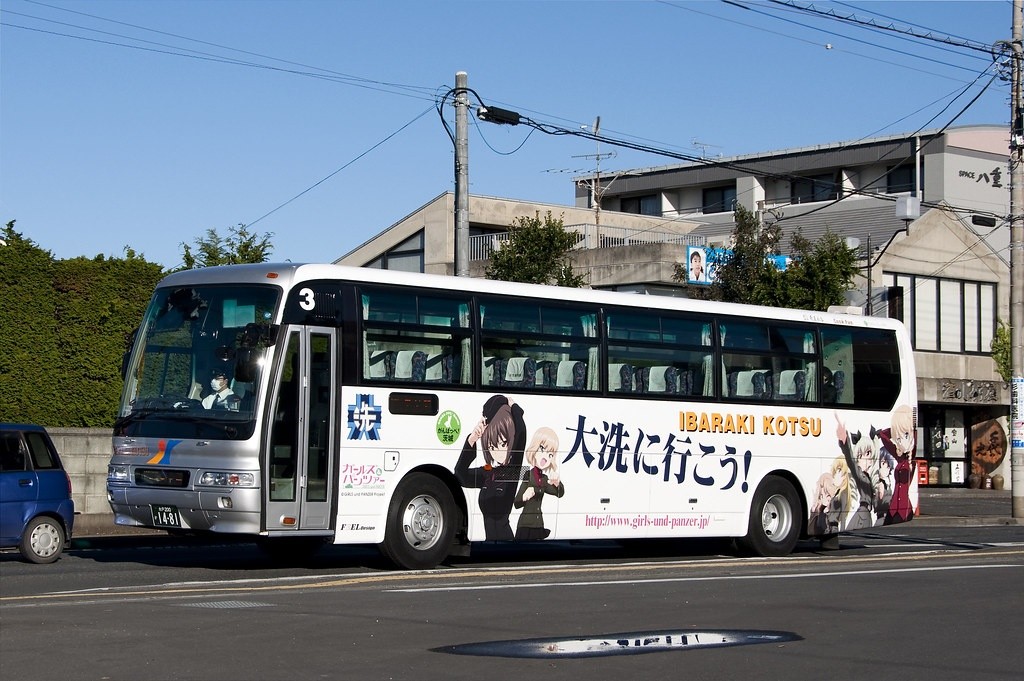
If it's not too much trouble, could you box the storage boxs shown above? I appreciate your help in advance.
[913,460,927,484]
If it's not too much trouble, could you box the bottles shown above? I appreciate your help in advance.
[992,474,1004,490]
[981,473,992,489]
[968,472,981,489]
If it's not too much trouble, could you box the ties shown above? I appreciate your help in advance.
[212,395,219,409]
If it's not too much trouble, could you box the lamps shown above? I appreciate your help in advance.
[962,378,974,387]
[996,381,1008,389]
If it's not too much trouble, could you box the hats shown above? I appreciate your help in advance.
[209,368,228,379]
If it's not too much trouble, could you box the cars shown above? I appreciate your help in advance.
[0,424,74,564]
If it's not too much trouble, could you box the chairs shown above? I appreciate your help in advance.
[289,353,329,402]
[368,349,845,403]
[0,438,24,470]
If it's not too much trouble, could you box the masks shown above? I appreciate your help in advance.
[211,379,226,391]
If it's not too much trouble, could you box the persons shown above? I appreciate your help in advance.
[944,436,949,449]
[689,251,706,282]
[823,366,837,403]
[174,366,237,409]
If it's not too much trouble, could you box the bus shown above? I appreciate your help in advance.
[104,263,922,567]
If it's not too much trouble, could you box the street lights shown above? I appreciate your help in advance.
[845,196,921,315]
